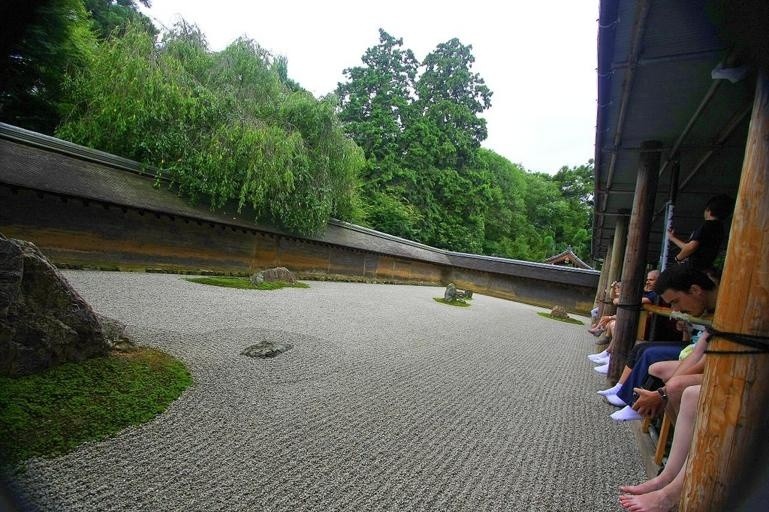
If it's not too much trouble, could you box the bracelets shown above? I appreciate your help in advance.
[675,255,681,261]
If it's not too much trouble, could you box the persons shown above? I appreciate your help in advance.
[666,198,727,269]
[585,262,726,511]
